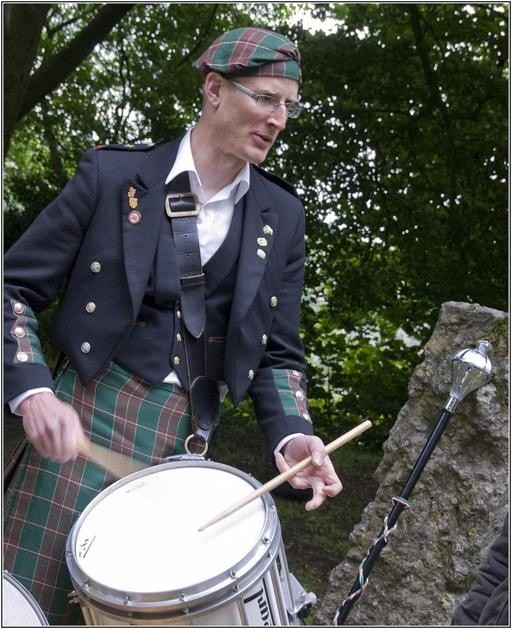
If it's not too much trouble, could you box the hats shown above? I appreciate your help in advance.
[192,27,304,84]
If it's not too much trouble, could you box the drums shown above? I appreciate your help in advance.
[3,571,50,626]
[65,458,316,626]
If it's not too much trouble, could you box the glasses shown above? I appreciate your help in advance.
[230,80,302,119]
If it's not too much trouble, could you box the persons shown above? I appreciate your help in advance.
[3,25,346,626]
